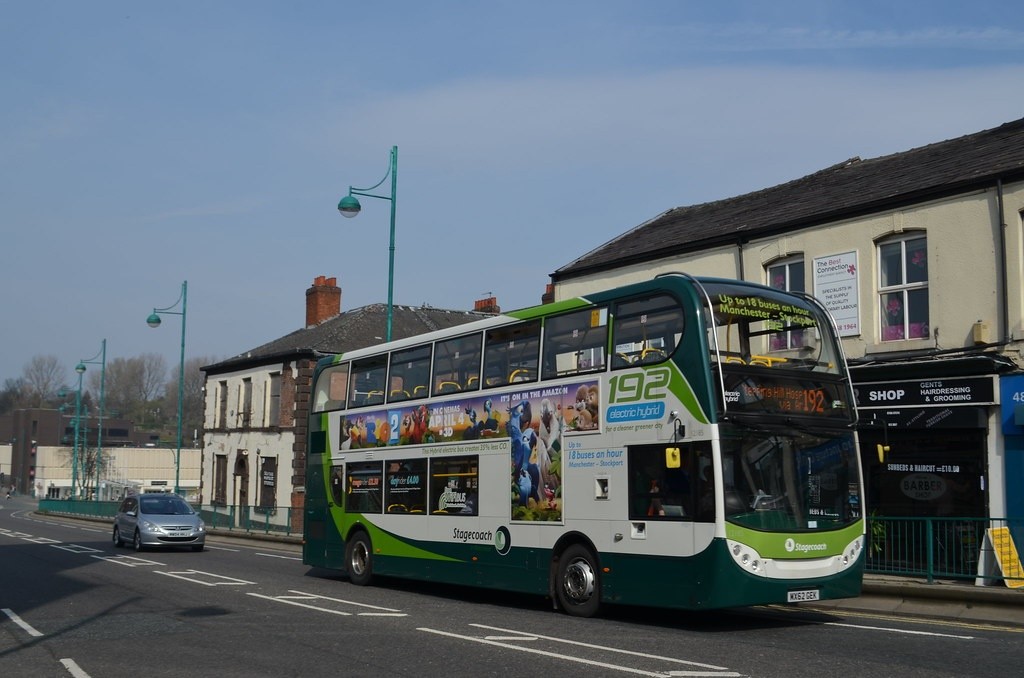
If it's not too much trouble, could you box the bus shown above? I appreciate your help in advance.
[300,271,868,617]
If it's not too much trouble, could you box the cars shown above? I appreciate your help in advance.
[111,491,207,553]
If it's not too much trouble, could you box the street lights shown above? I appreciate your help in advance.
[58,378,83,499]
[147,280,187,494]
[74,339,109,500]
[337,144,399,342]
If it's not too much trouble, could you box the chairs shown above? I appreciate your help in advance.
[388,504,423,514]
[323,348,668,412]
